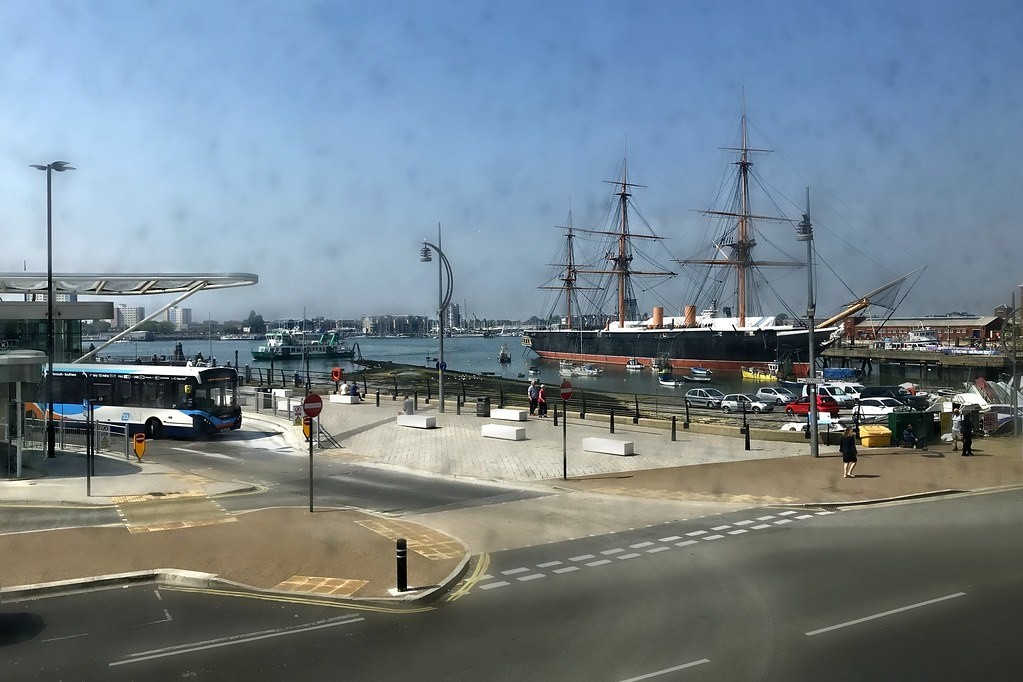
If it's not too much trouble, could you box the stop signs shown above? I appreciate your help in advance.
[304,419,310,425]
[560,380,573,401]
[136,434,145,444]
[304,394,323,417]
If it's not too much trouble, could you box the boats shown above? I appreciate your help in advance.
[250,322,356,359]
[898,382,963,399]
[625,336,713,388]
[740,364,863,388]
[528,364,541,376]
[497,341,511,363]
[481,371,502,378]
[559,319,604,378]
[425,350,438,361]
[873,321,941,366]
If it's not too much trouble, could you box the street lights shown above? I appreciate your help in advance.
[30,161,77,457]
[419,221,454,413]
[795,186,819,457]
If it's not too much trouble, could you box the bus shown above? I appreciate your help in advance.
[25,362,243,440]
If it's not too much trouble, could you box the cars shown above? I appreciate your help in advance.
[686,388,840,419]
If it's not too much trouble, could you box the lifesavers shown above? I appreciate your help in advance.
[331,367,342,382]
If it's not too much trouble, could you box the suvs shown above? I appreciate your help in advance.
[802,382,928,423]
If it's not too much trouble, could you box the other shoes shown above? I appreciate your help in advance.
[962,453,969,456]
[847,473,855,477]
[361,399,364,401]
[844,474,847,477]
[967,452,974,456]
[953,448,957,451]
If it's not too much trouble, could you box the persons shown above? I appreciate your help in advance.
[959,413,974,456]
[538,384,547,418]
[339,380,350,396]
[350,380,365,401]
[951,408,964,451]
[186,351,216,367]
[839,426,858,478]
[154,391,164,408]
[903,424,919,450]
[528,381,538,416]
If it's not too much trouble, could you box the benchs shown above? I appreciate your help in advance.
[490,409,527,420]
[583,437,634,455]
[482,424,526,440]
[272,389,300,411]
[217,395,247,406]
[396,415,436,428]
[330,394,359,404]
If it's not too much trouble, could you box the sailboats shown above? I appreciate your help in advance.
[521,85,928,376]
